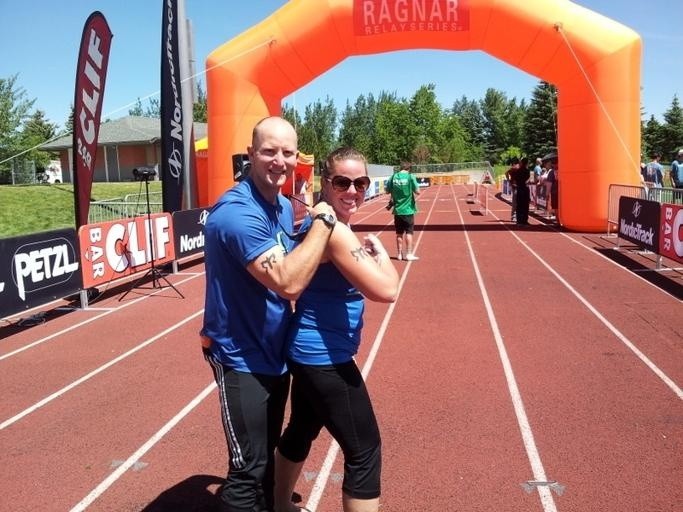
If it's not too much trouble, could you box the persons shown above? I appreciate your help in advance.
[272,148,401,511]
[199,115,340,511]
[385,160,420,261]
[294,173,314,195]
[504,150,683,227]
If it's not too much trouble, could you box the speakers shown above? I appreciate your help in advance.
[231,154,251,182]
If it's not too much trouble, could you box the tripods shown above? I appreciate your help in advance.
[119,184,186,300]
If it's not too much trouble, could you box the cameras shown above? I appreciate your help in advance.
[385,201,395,210]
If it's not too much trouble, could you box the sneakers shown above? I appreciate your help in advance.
[406,254,419,260]
[397,254,403,259]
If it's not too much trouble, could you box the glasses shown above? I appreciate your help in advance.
[325,175,370,192]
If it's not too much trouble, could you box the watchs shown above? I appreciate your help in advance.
[313,212,335,229]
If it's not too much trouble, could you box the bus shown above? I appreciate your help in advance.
[35,160,63,184]
